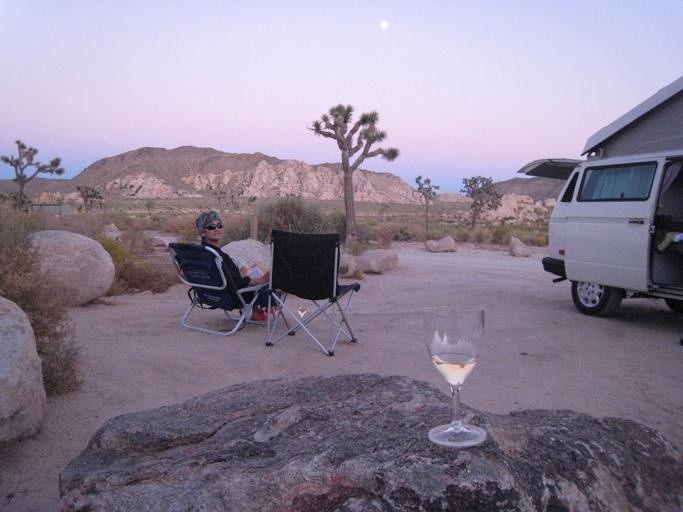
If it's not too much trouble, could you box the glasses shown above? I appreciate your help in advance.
[206,222,223,230]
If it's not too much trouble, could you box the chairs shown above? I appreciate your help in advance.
[264,228,361,356]
[166,239,281,338]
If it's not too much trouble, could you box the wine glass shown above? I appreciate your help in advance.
[422,305,487,444]
[296,300,307,328]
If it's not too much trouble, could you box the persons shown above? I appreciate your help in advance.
[193,209,279,321]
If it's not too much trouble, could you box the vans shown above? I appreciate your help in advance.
[516,150,682,317]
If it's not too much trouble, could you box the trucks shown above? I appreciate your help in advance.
[19,201,73,220]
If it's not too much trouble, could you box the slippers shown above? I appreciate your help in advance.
[265,305,280,314]
[251,310,268,320]
